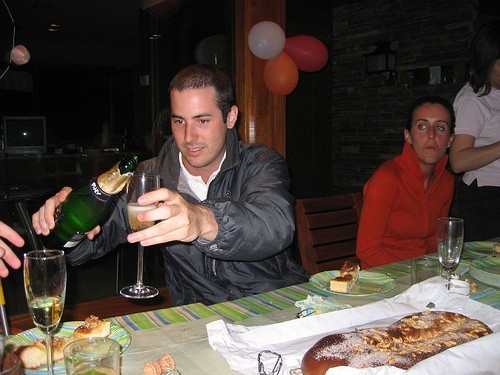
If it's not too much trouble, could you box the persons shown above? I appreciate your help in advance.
[355,96,455,270]
[0,221,25,277]
[447,21,500,213]
[31,65,312,305]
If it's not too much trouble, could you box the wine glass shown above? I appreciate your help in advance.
[435,217,464,291]
[119,174,160,298]
[23,249,68,375]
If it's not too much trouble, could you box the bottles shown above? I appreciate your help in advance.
[35,150,143,256]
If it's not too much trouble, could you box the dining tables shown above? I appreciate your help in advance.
[0,234,500,375]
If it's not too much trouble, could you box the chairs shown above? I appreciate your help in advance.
[295,195,362,277]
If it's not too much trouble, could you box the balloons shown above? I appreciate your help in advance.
[194,35,231,69]
[284,35,328,72]
[248,21,286,59]
[263,53,298,96]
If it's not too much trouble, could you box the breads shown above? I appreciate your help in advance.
[301,311,493,375]
[14,339,47,369]
[329,260,360,293]
[51,314,111,361]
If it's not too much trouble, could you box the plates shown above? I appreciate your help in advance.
[462,241,500,256]
[468,258,500,288]
[4,320,132,372]
[308,270,398,297]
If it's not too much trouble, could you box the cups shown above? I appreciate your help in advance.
[0,350,22,375]
[410,257,440,287]
[62,337,121,375]
